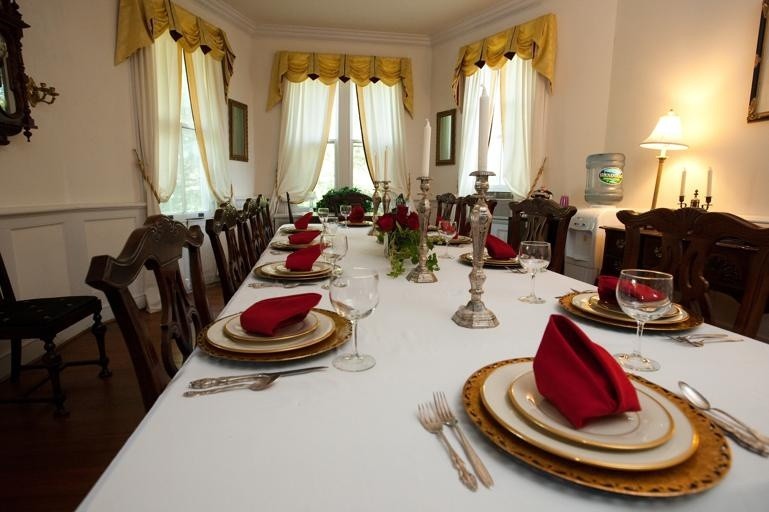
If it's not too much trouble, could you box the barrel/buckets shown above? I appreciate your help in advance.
[583,152,627,205]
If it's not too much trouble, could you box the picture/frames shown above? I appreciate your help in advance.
[743,0,769,126]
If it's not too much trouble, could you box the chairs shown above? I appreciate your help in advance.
[0,252,113,423]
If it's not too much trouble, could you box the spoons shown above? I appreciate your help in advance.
[673,374,764,447]
[182,371,284,401]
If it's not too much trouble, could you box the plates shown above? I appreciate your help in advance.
[268,239,332,250]
[428,233,473,245]
[281,226,318,234]
[250,261,343,281]
[197,304,353,362]
[340,220,373,227]
[456,357,731,500]
[456,249,518,268]
[552,290,706,335]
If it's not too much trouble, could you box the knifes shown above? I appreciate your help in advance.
[189,366,329,390]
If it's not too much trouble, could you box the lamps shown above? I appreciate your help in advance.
[26,75,61,109]
[637,106,693,227]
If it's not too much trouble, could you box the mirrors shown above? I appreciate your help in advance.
[226,97,251,162]
[434,104,457,167]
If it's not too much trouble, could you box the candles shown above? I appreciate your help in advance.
[678,168,687,198]
[373,152,380,182]
[704,167,714,196]
[473,81,492,173]
[383,143,391,182]
[421,116,431,179]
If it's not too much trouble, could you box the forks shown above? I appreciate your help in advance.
[416,387,494,498]
[663,330,745,349]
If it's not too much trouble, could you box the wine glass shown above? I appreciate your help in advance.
[615,268,675,374]
[317,206,331,230]
[514,238,555,305]
[318,231,348,291]
[435,220,458,260]
[327,264,382,373]
[339,204,352,230]
